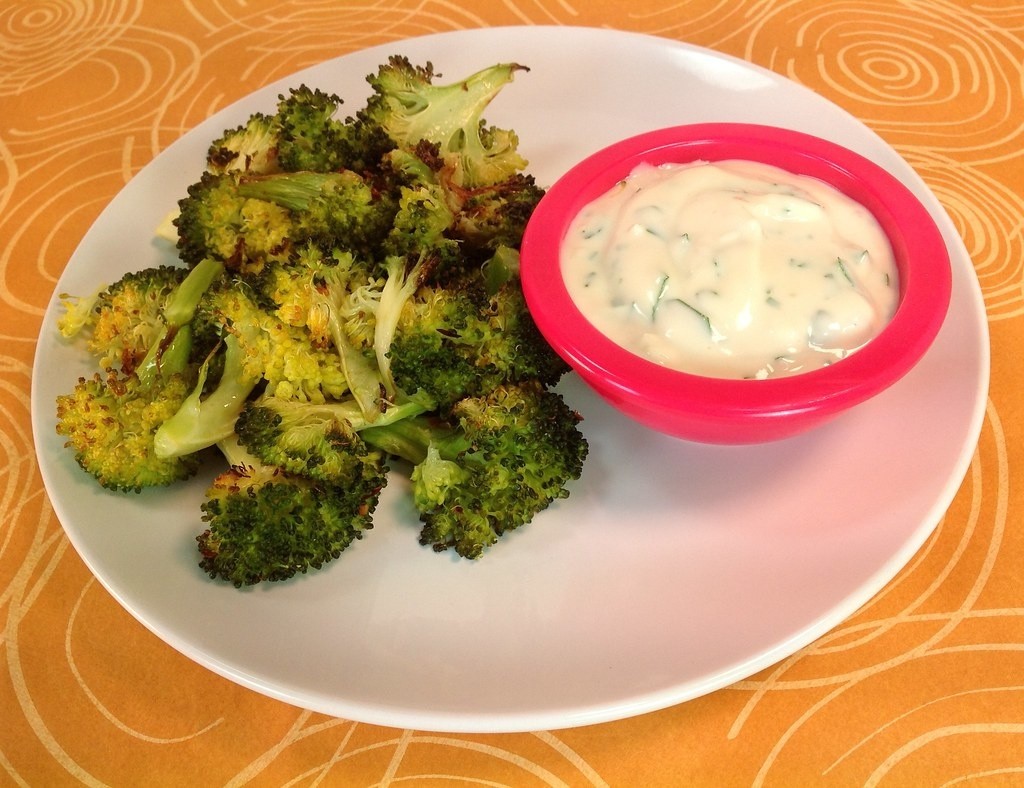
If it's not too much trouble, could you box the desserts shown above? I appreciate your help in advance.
[562,159,898,380]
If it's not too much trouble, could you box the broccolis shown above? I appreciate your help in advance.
[55,53,590,588]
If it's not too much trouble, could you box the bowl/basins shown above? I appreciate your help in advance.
[518,122,951,445]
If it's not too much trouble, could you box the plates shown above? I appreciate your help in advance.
[32,27,990,732]
